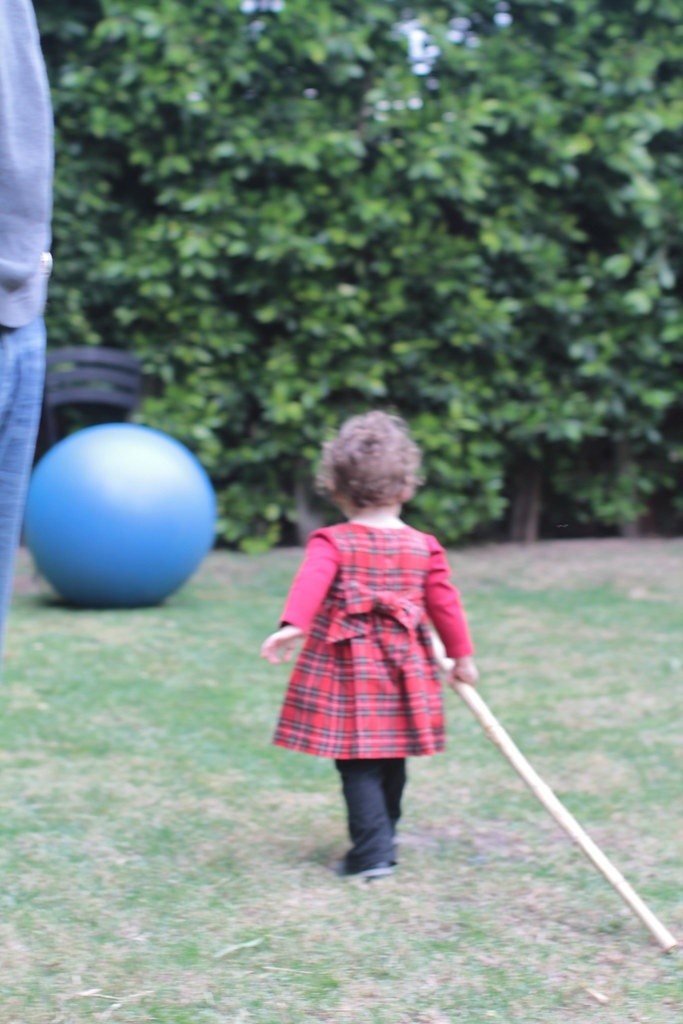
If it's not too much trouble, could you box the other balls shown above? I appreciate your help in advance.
[22,421,219,611]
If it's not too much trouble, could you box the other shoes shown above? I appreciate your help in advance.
[338,861,392,880]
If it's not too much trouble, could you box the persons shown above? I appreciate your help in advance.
[0,0,54,638]
[261,411,478,881]
